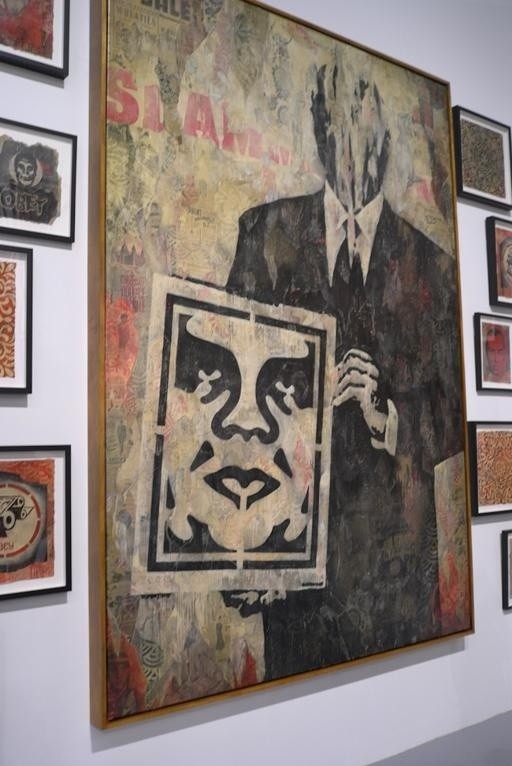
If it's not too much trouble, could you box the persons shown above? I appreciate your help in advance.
[482,327,510,384]
[499,236,511,287]
[0,152,59,224]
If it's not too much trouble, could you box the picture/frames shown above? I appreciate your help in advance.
[465,418,512,517]
[481,209,510,309]
[498,527,512,615]
[0,441,74,604]
[0,240,34,397]
[86,1,479,733]
[1,114,80,246]
[453,92,512,213]
[470,305,510,398]
[1,0,71,83]
